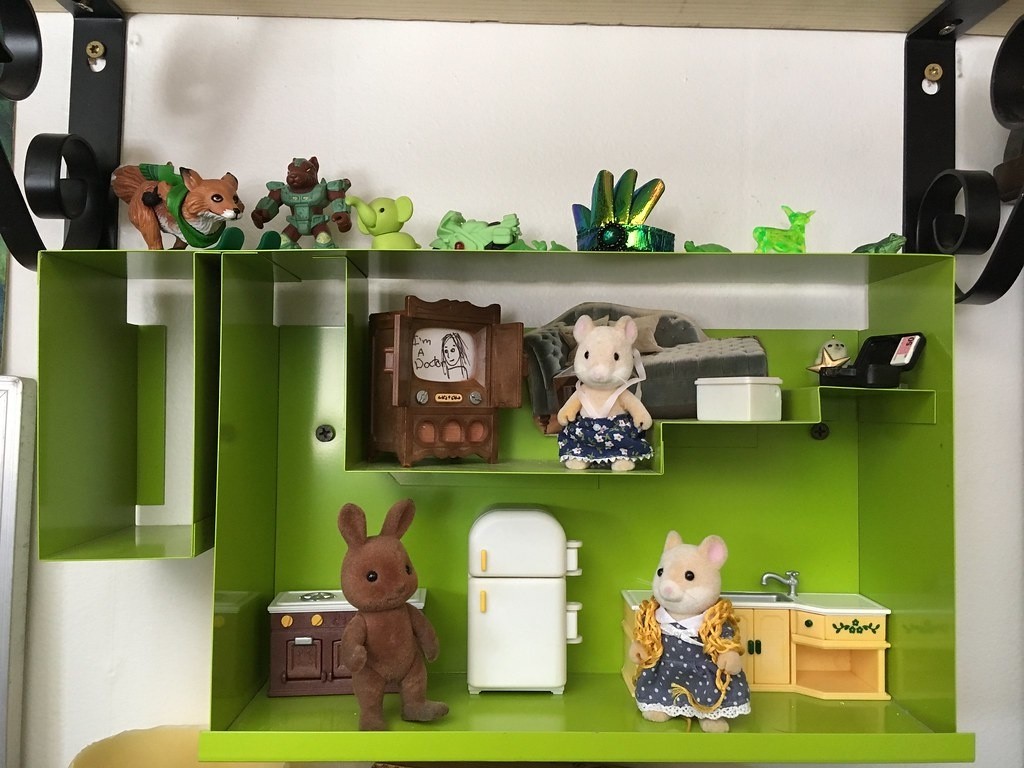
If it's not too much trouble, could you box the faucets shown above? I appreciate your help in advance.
[761,569,801,596]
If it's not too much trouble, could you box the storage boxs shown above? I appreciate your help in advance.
[693,376,785,421]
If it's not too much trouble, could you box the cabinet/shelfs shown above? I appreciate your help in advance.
[365,296,525,468]
[623,605,892,700]
[270,613,401,696]
[36,248,976,765]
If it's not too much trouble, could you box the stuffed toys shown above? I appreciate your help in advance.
[557,315,655,471]
[337,499,450,731]
[814,340,851,368]
[629,530,751,733]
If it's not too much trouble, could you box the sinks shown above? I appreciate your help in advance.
[721,592,789,603]
